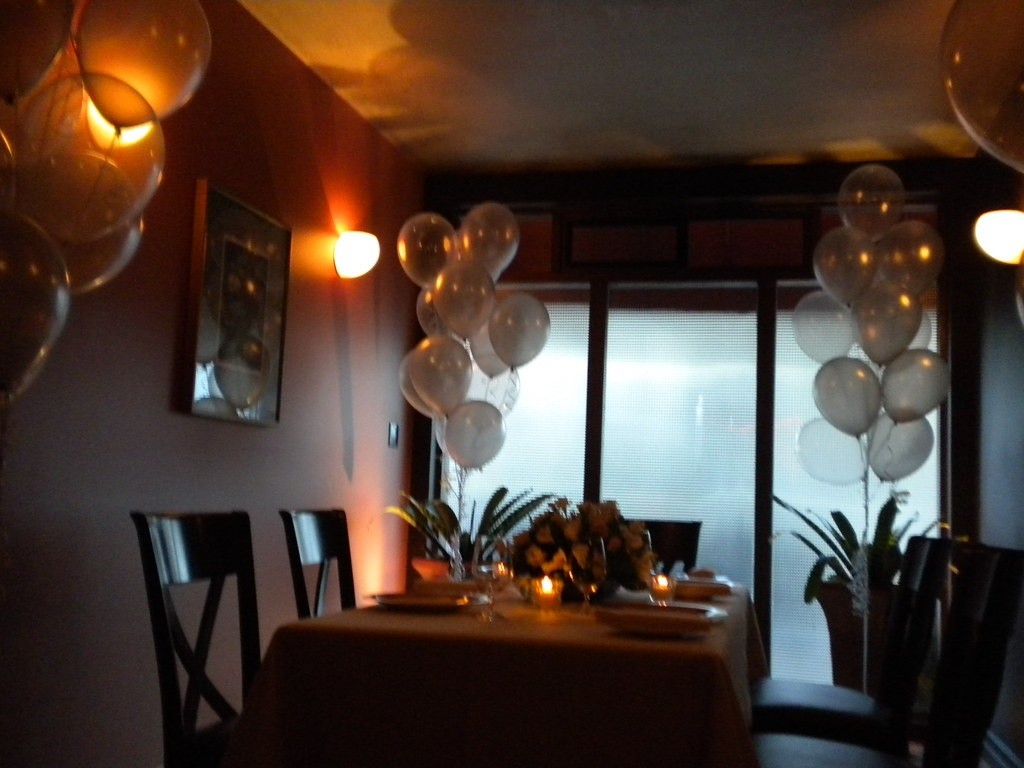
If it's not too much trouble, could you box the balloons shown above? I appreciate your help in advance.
[792,165,952,485]
[397,200,551,470]
[0,0,213,407]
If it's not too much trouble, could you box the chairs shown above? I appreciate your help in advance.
[130,509,261,768]
[278,509,356,620]
[750,536,953,759]
[754,545,1024,768]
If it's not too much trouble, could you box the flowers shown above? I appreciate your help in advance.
[493,498,655,593]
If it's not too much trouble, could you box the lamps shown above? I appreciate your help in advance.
[332,229,380,279]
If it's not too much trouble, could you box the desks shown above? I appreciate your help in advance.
[224,587,769,768]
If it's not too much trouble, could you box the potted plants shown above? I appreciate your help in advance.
[772,494,942,693]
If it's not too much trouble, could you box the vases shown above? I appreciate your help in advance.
[561,578,619,603]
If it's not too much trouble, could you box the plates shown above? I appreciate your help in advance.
[673,580,730,600]
[597,602,727,635]
[371,591,490,612]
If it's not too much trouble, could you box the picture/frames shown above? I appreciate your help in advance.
[175,177,292,428]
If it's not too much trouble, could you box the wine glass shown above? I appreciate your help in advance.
[568,536,605,615]
[472,536,511,625]
